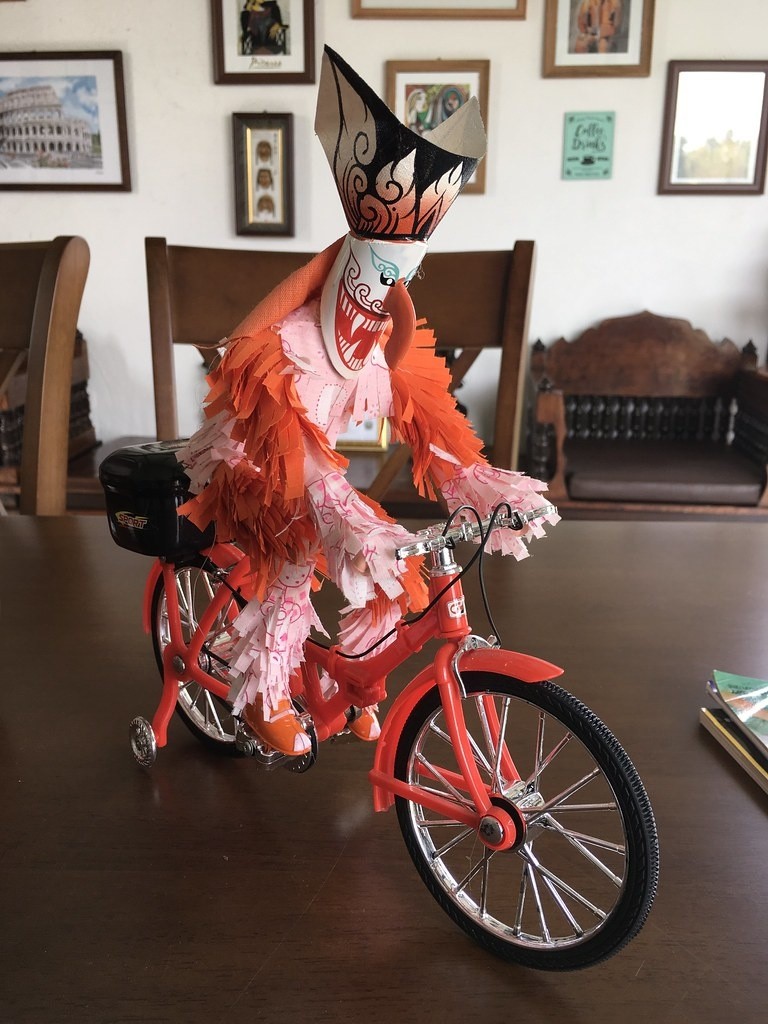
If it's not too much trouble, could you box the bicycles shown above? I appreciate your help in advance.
[98,437,660,976]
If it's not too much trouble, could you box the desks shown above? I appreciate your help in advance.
[0,515,768,1024]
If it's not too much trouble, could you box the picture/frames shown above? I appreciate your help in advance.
[0,50,132,193]
[350,0,528,21]
[210,0,317,86]
[385,56,491,195]
[232,111,295,238]
[658,60,768,196]
[542,0,656,78]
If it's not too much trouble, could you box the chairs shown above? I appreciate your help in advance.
[528,311,768,522]
[145,236,536,519]
[0,235,92,516]
